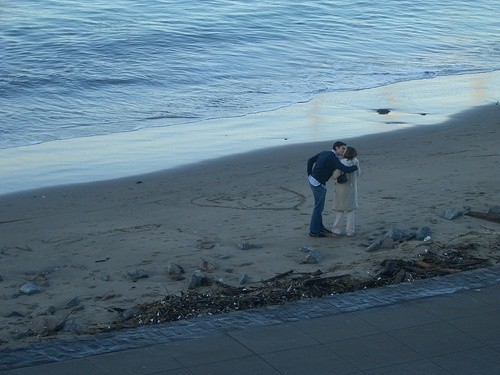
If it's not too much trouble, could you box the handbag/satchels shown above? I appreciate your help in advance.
[337,174,347,183]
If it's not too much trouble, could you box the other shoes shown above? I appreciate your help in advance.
[347,233,355,237]
[309,231,321,238]
[335,231,343,235]
[320,229,330,233]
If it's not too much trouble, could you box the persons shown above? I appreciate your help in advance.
[307,141,358,238]
[332,146,360,236]
